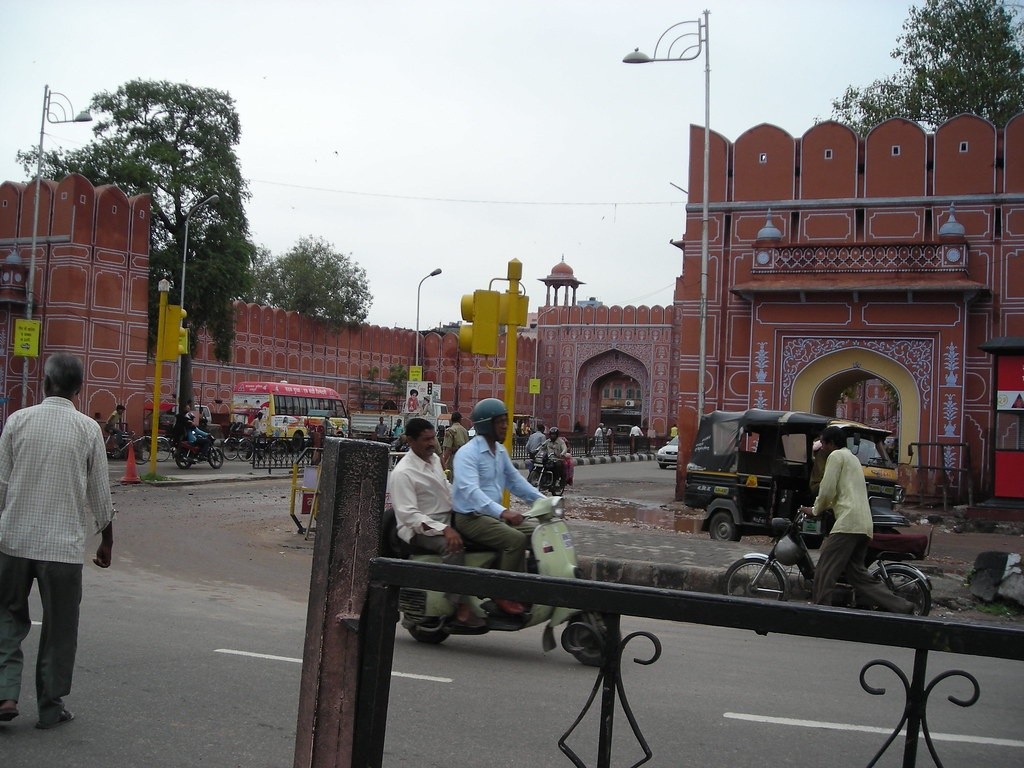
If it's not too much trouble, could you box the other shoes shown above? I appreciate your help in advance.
[198,454,208,461]
[909,603,922,615]
[497,597,524,614]
[451,611,486,628]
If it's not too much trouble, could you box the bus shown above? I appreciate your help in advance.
[231,380,350,452]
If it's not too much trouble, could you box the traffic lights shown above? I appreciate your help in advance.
[163,304,187,360]
[457,290,500,355]
[427,382,433,395]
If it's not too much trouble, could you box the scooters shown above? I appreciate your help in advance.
[720,504,932,617]
[387,495,621,668]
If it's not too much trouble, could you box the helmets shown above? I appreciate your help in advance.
[185,412,197,422]
[775,534,806,566]
[471,398,510,444]
[549,427,559,441]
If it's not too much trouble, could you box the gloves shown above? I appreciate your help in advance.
[208,433,212,436]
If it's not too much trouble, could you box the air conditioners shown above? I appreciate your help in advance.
[625,400,635,407]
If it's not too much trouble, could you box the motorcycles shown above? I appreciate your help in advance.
[682,408,912,543]
[390,431,409,467]
[527,445,565,496]
[173,435,224,469]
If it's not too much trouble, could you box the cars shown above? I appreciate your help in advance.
[656,434,678,469]
[602,424,633,444]
[144,411,177,438]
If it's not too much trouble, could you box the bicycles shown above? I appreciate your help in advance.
[105,430,171,465]
[223,432,287,462]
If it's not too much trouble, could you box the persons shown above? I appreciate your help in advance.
[245,412,263,461]
[671,423,678,441]
[0,350,115,730]
[375,417,387,435]
[591,423,612,456]
[387,418,487,629]
[574,421,586,444]
[242,399,260,406]
[525,424,573,487]
[796,426,917,615]
[392,418,404,465]
[322,415,336,436]
[512,421,518,435]
[629,423,644,454]
[451,397,548,616]
[400,432,446,471]
[407,388,434,416]
[281,416,289,435]
[175,405,213,461]
[443,412,470,483]
[104,405,126,458]
[199,408,206,422]
[521,419,529,434]
[182,399,196,411]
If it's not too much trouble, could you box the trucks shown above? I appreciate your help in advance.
[350,402,450,462]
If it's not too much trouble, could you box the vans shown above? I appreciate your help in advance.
[172,404,211,424]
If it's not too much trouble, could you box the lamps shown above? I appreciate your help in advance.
[757,207,783,239]
[939,201,965,238]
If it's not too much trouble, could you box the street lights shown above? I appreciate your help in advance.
[21,83,93,408]
[621,8,709,421]
[532,307,556,420]
[174,194,220,426]
[415,268,442,382]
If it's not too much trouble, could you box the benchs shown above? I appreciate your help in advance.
[380,509,499,569]
[738,451,775,476]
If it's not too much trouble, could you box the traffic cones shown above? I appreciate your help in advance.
[120,440,142,484]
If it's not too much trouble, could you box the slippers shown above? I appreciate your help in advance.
[37,709,74,729]
[0,709,18,721]
